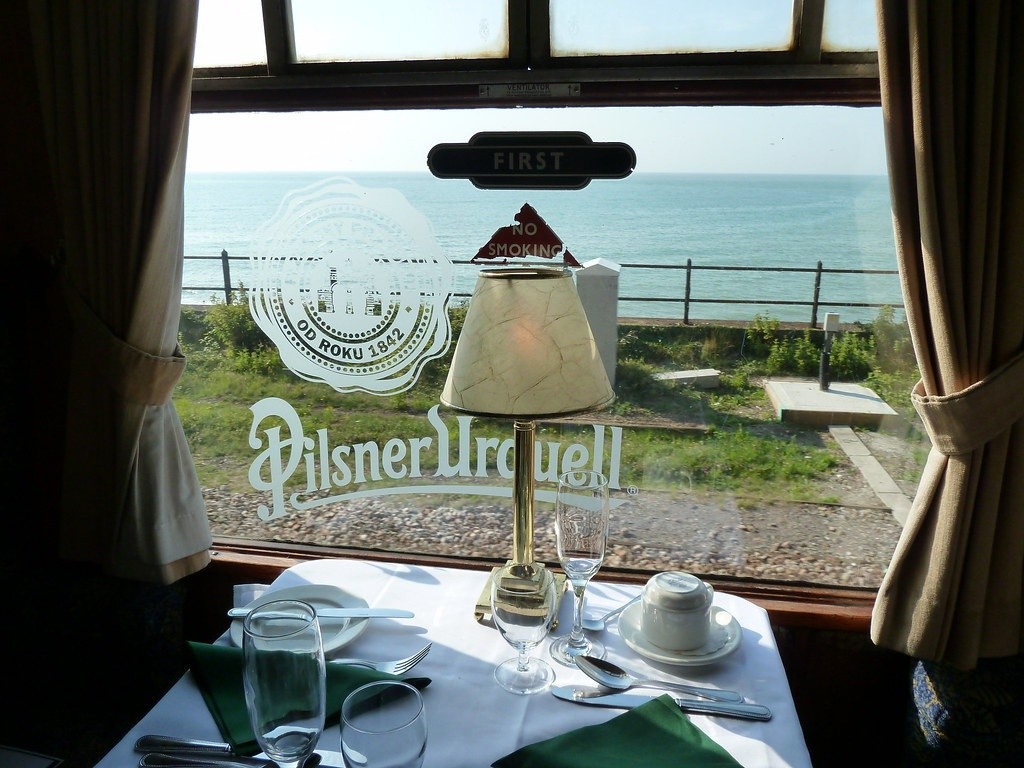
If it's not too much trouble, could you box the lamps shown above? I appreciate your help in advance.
[439,268,617,629]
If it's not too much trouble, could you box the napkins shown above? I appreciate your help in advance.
[186,640,433,758]
[490,694,745,768]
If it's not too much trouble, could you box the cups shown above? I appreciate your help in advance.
[641,570,714,650]
[340,681,428,768]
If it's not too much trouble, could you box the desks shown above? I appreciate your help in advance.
[95,558,813,768]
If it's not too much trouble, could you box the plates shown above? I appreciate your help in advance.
[618,600,743,663]
[231,585,370,653]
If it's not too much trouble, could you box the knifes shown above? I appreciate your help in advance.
[227,606,416,619]
[552,685,773,723]
[134,735,345,768]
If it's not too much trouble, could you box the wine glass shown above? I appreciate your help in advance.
[548,471,609,669]
[491,564,556,695]
[242,600,326,768]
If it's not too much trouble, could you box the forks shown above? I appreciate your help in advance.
[330,642,431,675]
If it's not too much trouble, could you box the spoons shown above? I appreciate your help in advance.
[576,655,744,705]
[140,752,321,768]
[579,594,640,631]
